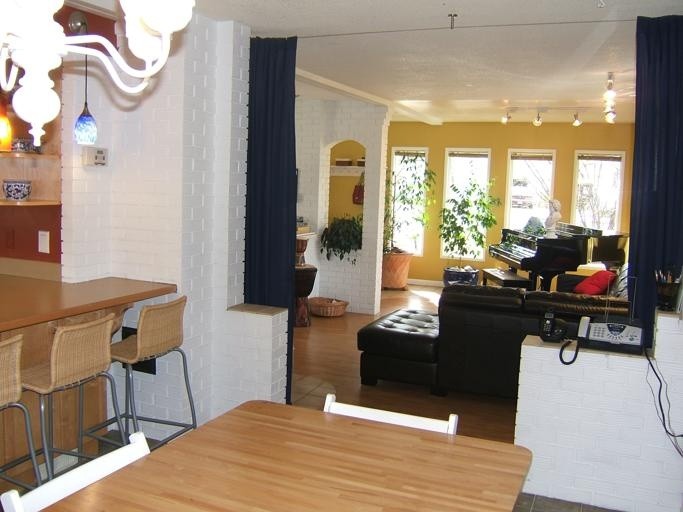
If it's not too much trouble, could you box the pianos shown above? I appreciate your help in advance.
[489,222,628,292]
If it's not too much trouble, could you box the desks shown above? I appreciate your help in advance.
[38,399,534,511]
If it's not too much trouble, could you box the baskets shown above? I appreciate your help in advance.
[306,296,349,317]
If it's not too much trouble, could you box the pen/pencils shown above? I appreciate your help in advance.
[654,269,683,312]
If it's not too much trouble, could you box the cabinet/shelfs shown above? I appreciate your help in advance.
[1,151,60,207]
[4,308,108,479]
[1,206,60,263]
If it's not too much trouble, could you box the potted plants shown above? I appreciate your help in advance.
[381,148,438,291]
[435,177,503,288]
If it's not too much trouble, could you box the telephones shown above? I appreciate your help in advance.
[540,307,568,343]
[577,313,645,355]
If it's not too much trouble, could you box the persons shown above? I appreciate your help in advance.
[544,198,561,238]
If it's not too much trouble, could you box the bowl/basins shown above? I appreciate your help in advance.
[12,137,46,153]
[2,179,32,202]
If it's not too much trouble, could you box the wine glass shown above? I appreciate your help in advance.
[656,281,679,312]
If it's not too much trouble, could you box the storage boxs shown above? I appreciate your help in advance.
[336,159,353,166]
[355,158,365,166]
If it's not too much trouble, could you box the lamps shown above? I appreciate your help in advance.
[66,11,98,145]
[0,0,196,147]
[500,73,616,127]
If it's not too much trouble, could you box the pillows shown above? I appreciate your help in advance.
[574,269,617,295]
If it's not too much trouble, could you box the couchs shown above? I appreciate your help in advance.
[437,263,632,399]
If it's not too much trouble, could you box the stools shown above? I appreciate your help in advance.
[356,307,439,386]
[481,267,529,291]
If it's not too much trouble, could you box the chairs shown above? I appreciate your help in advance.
[1,333,42,491]
[111,296,196,452]
[0,430,151,512]
[21,312,128,481]
[324,393,459,435]
